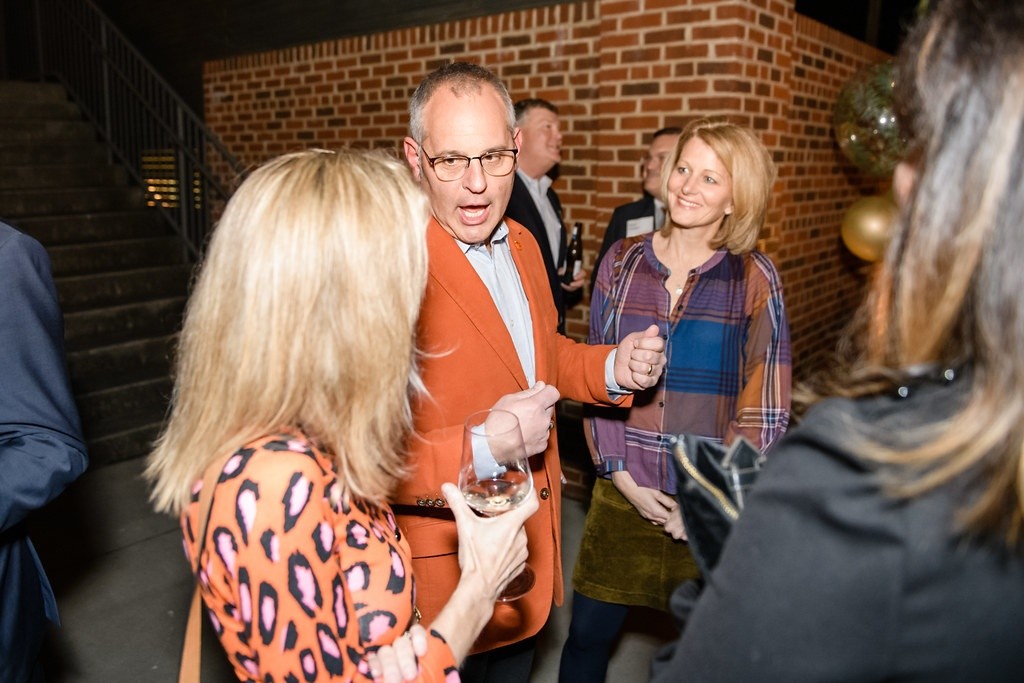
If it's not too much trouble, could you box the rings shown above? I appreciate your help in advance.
[549,420,554,430]
[646,363,653,376]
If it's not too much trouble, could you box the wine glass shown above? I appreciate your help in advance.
[458,409,536,602]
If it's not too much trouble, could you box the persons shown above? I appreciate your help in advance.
[141,147,539,683]
[663,0,1024,683]
[550,118,793,683]
[587,126,696,337]
[0,217,85,683]
[379,60,669,683]
[495,97,587,343]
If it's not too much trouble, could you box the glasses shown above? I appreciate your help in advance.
[421,141,518,181]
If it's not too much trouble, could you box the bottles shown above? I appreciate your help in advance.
[562,222,584,284]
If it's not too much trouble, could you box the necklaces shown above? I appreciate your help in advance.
[657,236,699,296]
